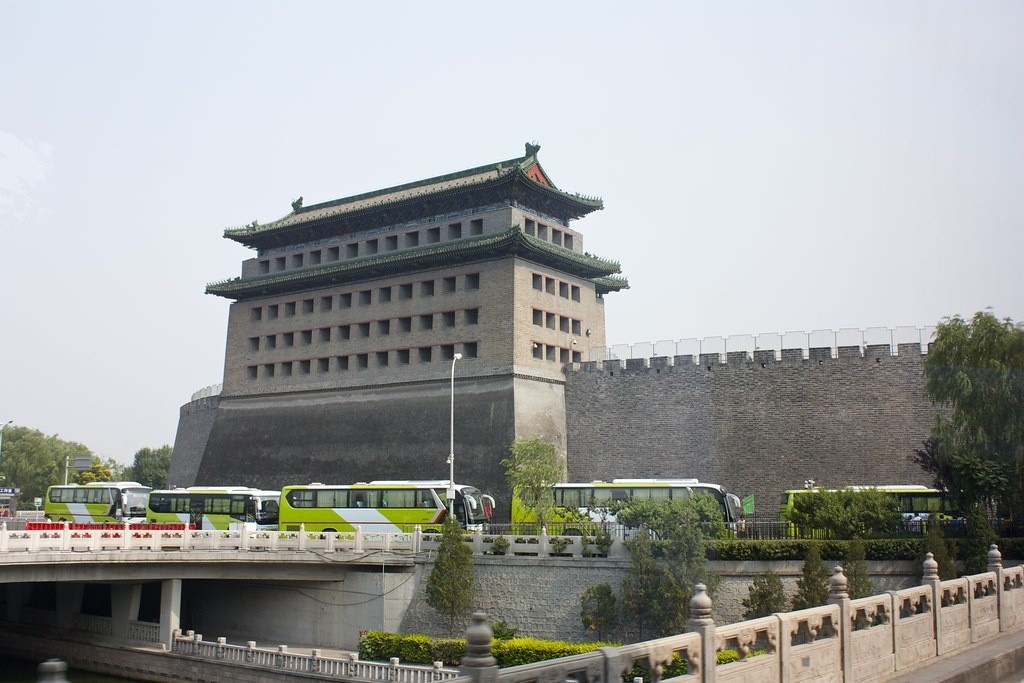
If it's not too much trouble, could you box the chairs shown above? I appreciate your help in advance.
[83,498,86,502]
[95,498,98,502]
[356,501,364,507]
[382,500,388,507]
[293,497,299,507]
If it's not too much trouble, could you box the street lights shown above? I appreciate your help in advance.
[447,352,463,526]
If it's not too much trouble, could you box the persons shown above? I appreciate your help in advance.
[906,512,922,532]
[737,516,746,538]
[940,514,944,529]
[44,514,53,551]
[955,513,967,532]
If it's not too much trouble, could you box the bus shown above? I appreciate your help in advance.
[146,484,280,552]
[279,480,496,556]
[780,480,967,539]
[45,481,153,524]
[511,477,742,542]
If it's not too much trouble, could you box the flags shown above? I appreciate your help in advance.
[742,495,754,515]
